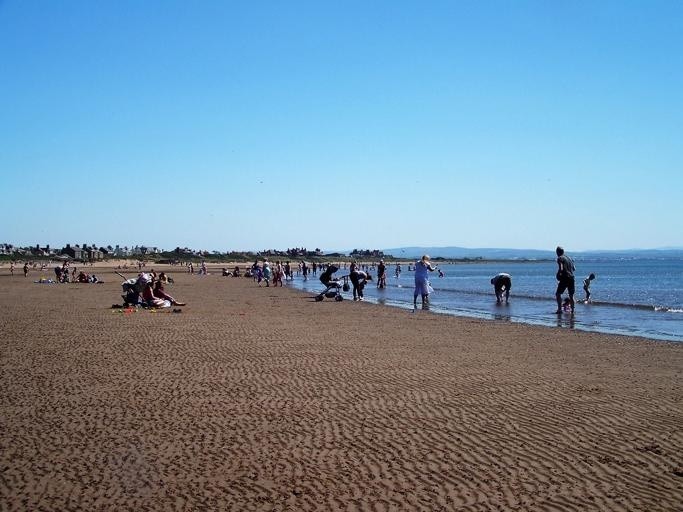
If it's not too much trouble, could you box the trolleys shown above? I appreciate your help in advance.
[314,274,351,301]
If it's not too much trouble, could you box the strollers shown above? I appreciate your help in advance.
[113,269,149,309]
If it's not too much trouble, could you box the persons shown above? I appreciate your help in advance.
[358,279,369,299]
[5,255,104,284]
[561,297,573,315]
[112,255,217,309]
[554,246,576,315]
[499,286,506,303]
[581,272,596,301]
[220,255,463,288]
[489,272,512,305]
[425,278,433,303]
[411,254,439,305]
[349,270,373,302]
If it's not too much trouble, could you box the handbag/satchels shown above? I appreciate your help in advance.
[343,283,350,291]
[335,294,343,301]
